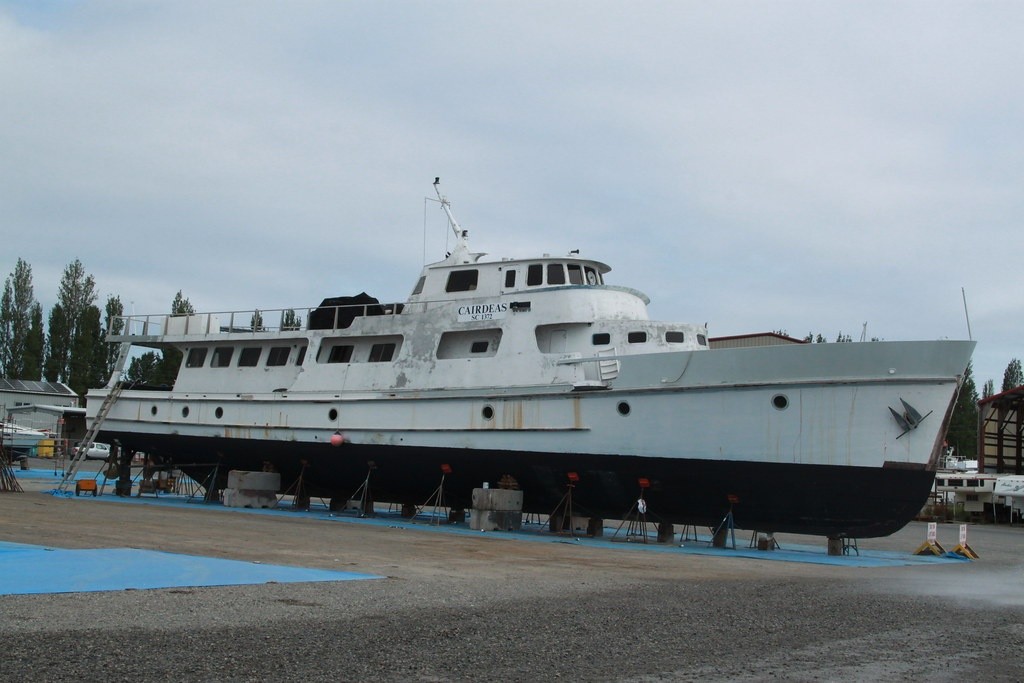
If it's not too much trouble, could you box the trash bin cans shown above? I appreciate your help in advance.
[19,455,28,470]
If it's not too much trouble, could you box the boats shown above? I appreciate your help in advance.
[83,171,978,541]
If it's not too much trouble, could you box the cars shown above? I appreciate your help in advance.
[71,442,111,463]
